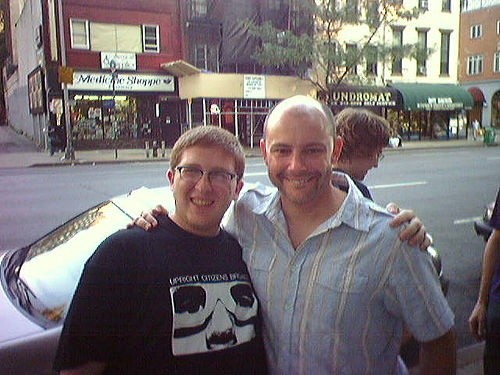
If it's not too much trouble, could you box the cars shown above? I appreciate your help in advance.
[1,184,448,375]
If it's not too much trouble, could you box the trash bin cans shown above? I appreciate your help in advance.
[485,127,496,143]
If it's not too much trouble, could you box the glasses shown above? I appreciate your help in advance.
[173,166,240,186]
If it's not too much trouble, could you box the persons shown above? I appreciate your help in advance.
[51,125,434,375]
[472,119,480,141]
[323,108,392,206]
[470,181,500,373]
[127,95,459,374]
